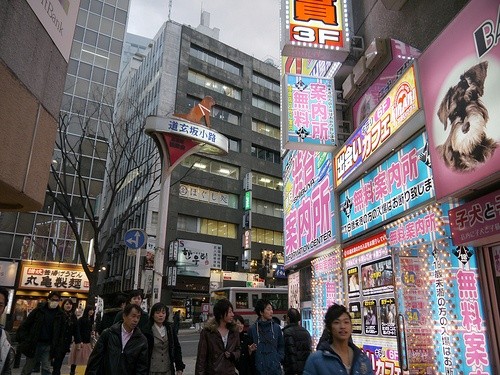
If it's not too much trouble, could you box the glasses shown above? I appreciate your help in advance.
[49,298,59,302]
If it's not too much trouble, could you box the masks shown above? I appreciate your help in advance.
[49,301,58,308]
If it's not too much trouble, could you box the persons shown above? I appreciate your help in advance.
[280,308,311,375]
[231,314,257,375]
[195,298,241,375]
[172,309,181,334]
[85,304,149,375]
[304,303,373,375]
[0,285,16,375]
[47,299,73,375]
[363,263,393,288]
[380,305,393,323]
[69,305,95,375]
[366,309,376,325]
[244,299,282,375]
[19,290,65,375]
[115,296,152,340]
[348,274,359,290]
[146,302,186,375]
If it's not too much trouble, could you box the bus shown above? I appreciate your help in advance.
[207,285,289,327]
